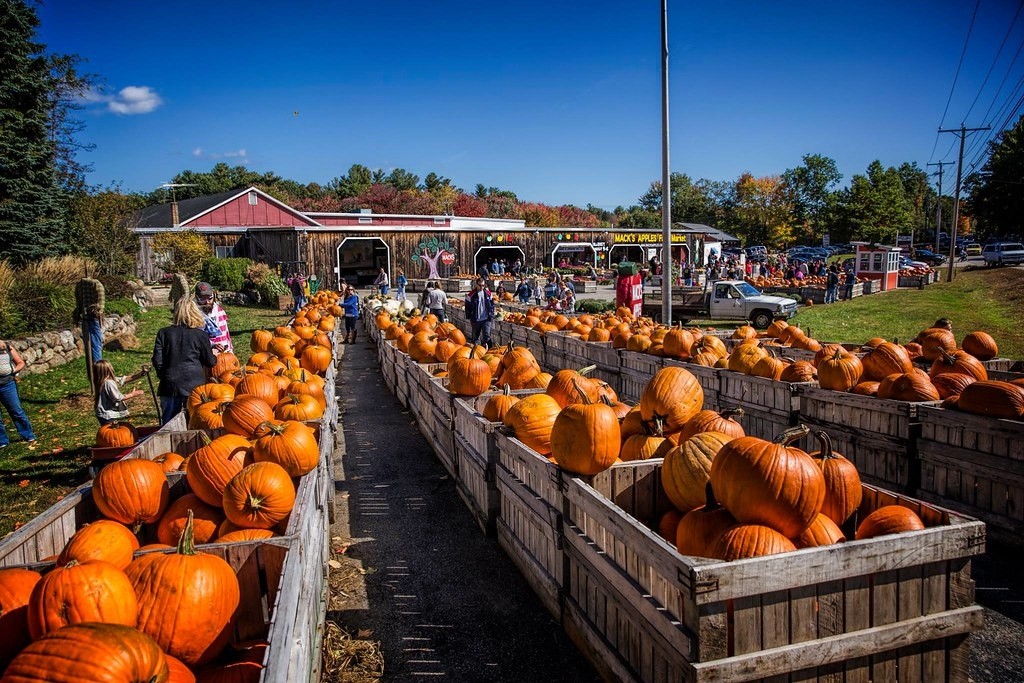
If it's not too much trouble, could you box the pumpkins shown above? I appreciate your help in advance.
[0,290,342,683]
[363,268,1024,653]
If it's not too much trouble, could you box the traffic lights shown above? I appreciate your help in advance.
[486,235,513,243]
[557,233,580,241]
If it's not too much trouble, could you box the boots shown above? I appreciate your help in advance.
[340,332,351,343]
[349,328,357,345]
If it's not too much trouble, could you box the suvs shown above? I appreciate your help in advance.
[860,250,947,273]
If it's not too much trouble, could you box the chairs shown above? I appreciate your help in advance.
[715,288,722,299]
[724,287,733,299]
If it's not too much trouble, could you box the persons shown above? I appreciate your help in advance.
[613,249,855,305]
[910,244,967,263]
[0,283,231,449]
[287,256,603,351]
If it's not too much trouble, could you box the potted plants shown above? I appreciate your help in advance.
[265,273,291,311]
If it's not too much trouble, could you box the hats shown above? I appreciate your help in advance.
[195,282,212,296]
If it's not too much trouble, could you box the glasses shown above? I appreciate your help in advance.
[347,288,353,291]
[196,292,213,302]
[478,284,485,286]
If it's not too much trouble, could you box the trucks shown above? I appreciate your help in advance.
[641,280,798,329]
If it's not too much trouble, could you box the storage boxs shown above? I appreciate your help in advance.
[0,270,1024,683]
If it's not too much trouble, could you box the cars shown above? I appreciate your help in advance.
[730,246,768,257]
[939,233,982,255]
[778,245,855,265]
[982,242,1024,266]
